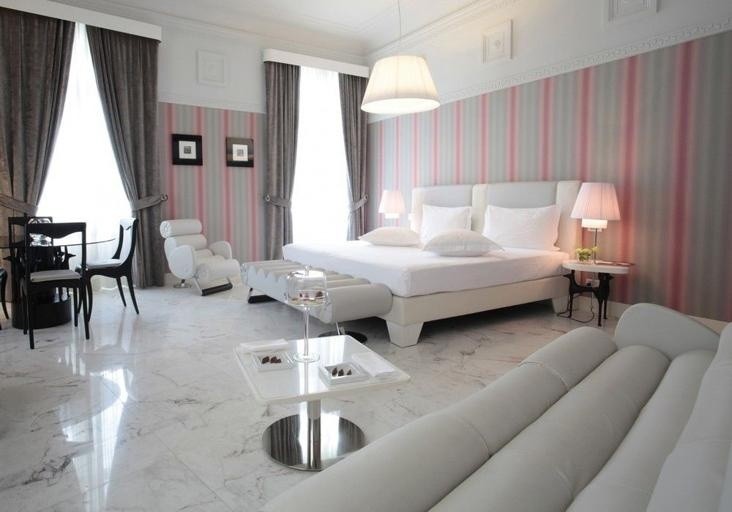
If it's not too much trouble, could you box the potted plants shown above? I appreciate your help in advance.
[575,246,598,264]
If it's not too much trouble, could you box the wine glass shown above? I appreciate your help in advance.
[286,271,327,362]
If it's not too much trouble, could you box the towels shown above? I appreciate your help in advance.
[241,338,289,352]
[352,352,398,378]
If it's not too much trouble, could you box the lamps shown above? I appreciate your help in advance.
[571,182,621,263]
[379,190,404,226]
[359,0,440,115]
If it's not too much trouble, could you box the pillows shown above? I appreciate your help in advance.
[359,226,422,246]
[422,228,505,258]
[422,204,472,235]
[482,205,559,251]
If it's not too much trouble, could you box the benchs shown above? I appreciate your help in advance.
[242,260,392,343]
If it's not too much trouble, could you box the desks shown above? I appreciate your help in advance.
[233,336,410,472]
[561,260,629,327]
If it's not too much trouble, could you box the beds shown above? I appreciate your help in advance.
[281,180,581,348]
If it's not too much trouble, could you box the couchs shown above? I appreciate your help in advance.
[259,302,732,512]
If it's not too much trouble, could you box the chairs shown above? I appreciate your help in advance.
[1,215,140,349]
[160,219,240,295]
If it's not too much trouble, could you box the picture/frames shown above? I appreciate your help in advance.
[198,51,226,87]
[601,0,658,30]
[172,134,203,165]
[226,136,254,168]
[482,19,513,64]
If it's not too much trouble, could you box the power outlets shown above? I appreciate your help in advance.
[585,278,600,288]
